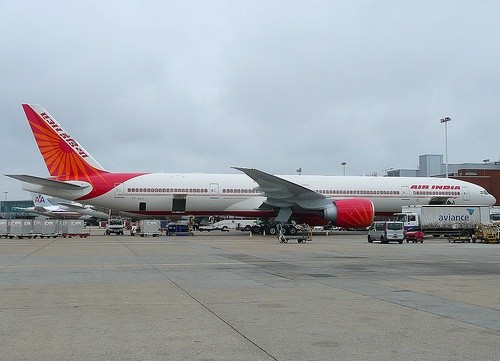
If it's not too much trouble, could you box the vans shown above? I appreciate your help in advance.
[366,220,405,244]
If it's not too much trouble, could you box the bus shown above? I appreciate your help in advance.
[208,215,262,231]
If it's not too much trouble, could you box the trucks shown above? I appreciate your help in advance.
[105,218,125,235]
[392,205,490,237]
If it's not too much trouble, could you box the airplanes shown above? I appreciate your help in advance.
[2,104,497,237]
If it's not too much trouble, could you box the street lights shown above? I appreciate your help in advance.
[340,161,346,176]
[296,168,302,175]
[4,191,8,201]
[440,116,451,178]
